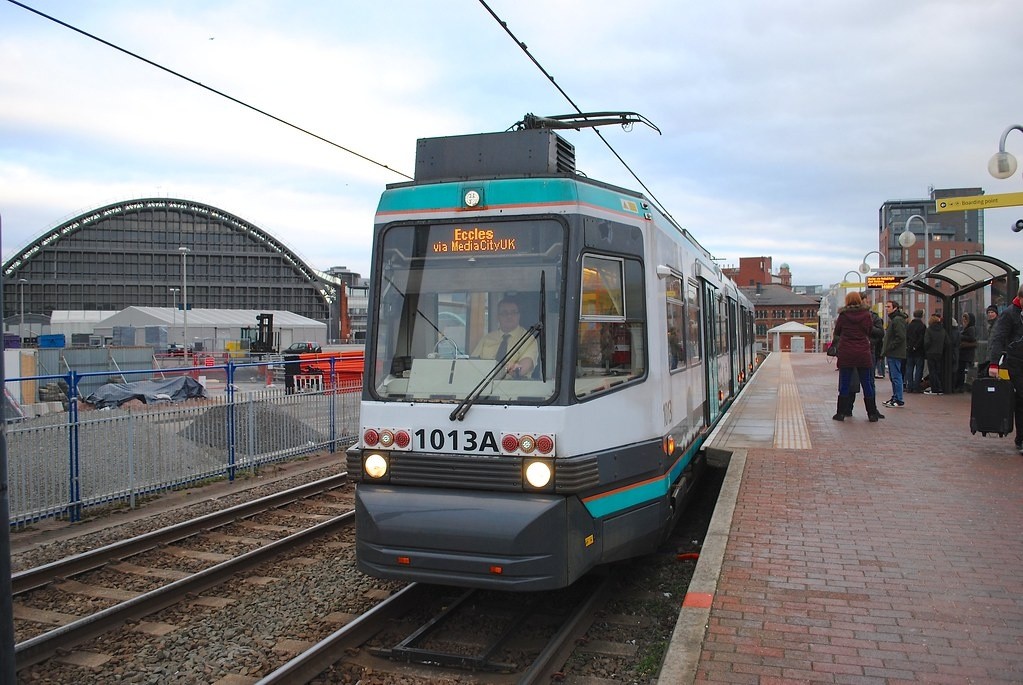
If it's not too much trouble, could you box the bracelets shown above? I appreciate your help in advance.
[514,366,520,378]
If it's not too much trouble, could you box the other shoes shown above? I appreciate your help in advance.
[1015,444,1023,453]
[876,409,885,419]
[875,374,884,379]
[846,411,852,417]
[924,389,943,395]
[903,388,913,393]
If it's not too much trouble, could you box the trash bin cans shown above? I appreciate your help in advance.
[283,355,302,395]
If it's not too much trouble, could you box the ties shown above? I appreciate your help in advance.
[495,334,511,380]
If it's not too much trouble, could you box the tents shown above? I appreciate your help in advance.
[49,310,131,348]
[93,305,327,362]
[766,321,818,353]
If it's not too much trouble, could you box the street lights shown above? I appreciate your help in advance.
[898,215,929,329]
[841,271,862,293]
[178,246,191,367]
[169,288,180,344]
[18,278,27,336]
[859,251,887,334]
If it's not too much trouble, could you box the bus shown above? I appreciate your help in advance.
[346,111,758,593]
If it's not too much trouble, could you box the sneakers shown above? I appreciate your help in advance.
[883,396,896,406]
[886,399,905,408]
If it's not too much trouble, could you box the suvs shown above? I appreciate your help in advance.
[281,341,321,360]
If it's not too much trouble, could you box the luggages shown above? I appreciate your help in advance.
[969,375,1014,438]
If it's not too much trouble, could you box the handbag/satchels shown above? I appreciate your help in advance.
[826,335,840,357]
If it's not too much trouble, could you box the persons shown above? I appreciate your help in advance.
[843,293,1001,419]
[468,299,539,381]
[989,284,1023,455]
[831,292,879,422]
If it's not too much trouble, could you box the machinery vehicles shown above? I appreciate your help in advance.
[225,313,281,361]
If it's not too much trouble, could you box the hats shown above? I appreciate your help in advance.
[986,304,998,316]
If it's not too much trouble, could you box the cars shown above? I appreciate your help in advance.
[167,342,192,357]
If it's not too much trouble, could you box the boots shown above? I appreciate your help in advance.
[863,397,878,422]
[833,395,848,421]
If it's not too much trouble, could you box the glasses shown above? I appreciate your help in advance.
[499,310,520,317]
[884,305,890,308]
